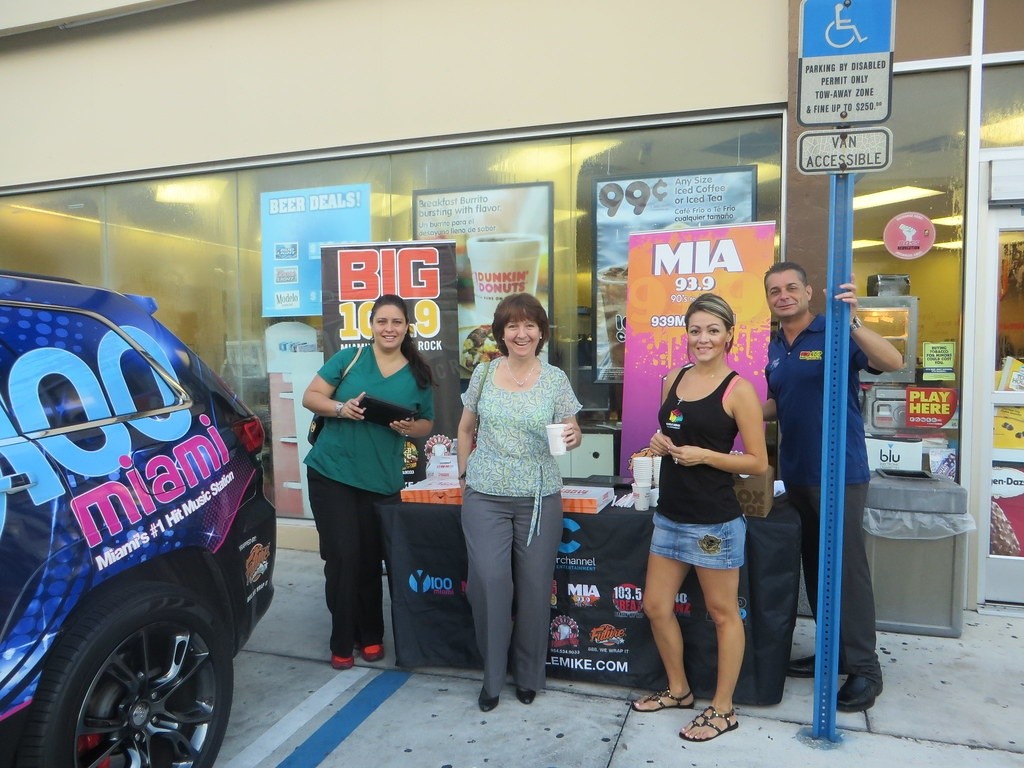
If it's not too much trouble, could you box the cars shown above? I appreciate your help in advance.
[1,269,280,768]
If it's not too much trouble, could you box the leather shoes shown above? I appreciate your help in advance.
[786,656,815,678]
[516,688,536,704]
[836,673,883,712]
[478,685,499,712]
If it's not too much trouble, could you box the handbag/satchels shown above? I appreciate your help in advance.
[308,347,362,446]
[357,397,417,427]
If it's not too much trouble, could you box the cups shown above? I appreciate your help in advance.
[596,263,627,366]
[631,456,662,511]
[466,232,544,324]
[546,424,567,455]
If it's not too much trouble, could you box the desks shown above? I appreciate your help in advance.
[375,480,801,708]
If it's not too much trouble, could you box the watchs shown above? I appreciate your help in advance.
[850,316,863,331]
[336,402,345,418]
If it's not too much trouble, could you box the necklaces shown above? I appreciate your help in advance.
[506,357,537,386]
[677,367,716,406]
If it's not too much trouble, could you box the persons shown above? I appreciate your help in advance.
[761,260,903,712]
[458,293,584,714]
[642,292,768,739]
[301,293,435,671]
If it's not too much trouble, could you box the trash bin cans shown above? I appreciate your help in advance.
[861,468,969,639]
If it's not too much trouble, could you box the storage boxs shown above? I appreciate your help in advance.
[732,464,775,518]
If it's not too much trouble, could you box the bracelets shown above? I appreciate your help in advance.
[459,471,466,480]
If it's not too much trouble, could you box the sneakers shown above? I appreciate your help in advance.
[356,640,383,661]
[331,655,353,669]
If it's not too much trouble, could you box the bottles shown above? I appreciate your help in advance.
[451,438,458,455]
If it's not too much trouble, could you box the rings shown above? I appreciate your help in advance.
[405,435,409,437]
[675,458,679,465]
[402,429,406,434]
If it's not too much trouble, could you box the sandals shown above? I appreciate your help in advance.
[631,684,694,711]
[679,706,738,742]
